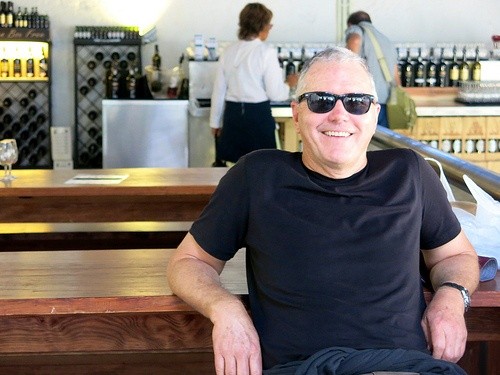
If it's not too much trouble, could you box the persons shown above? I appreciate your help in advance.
[210,2,299,167]
[344,12,401,130]
[166,43,479,375]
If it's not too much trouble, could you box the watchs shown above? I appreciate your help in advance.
[440,282,471,314]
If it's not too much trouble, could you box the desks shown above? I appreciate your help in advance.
[0,168,500,375]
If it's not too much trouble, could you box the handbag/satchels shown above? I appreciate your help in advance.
[386,85,416,130]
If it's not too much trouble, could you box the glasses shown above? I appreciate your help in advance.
[298,91,375,115]
[264,23,273,29]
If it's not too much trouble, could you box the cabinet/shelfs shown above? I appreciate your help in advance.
[0,26,53,170]
[74,38,142,169]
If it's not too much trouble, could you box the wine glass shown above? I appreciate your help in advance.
[0,139,19,180]
[457,80,500,103]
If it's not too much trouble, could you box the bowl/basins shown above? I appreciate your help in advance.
[146,66,184,100]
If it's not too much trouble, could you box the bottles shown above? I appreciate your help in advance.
[74,25,140,39]
[414,48,426,87]
[418,139,500,154]
[402,48,414,87]
[395,48,402,87]
[471,46,482,81]
[438,47,449,87]
[0,41,48,77]
[426,47,438,87]
[77,43,188,169]
[0,1,49,29]
[0,90,50,169]
[449,46,460,87]
[276,46,317,78]
[192,34,217,61]
[459,46,471,88]
